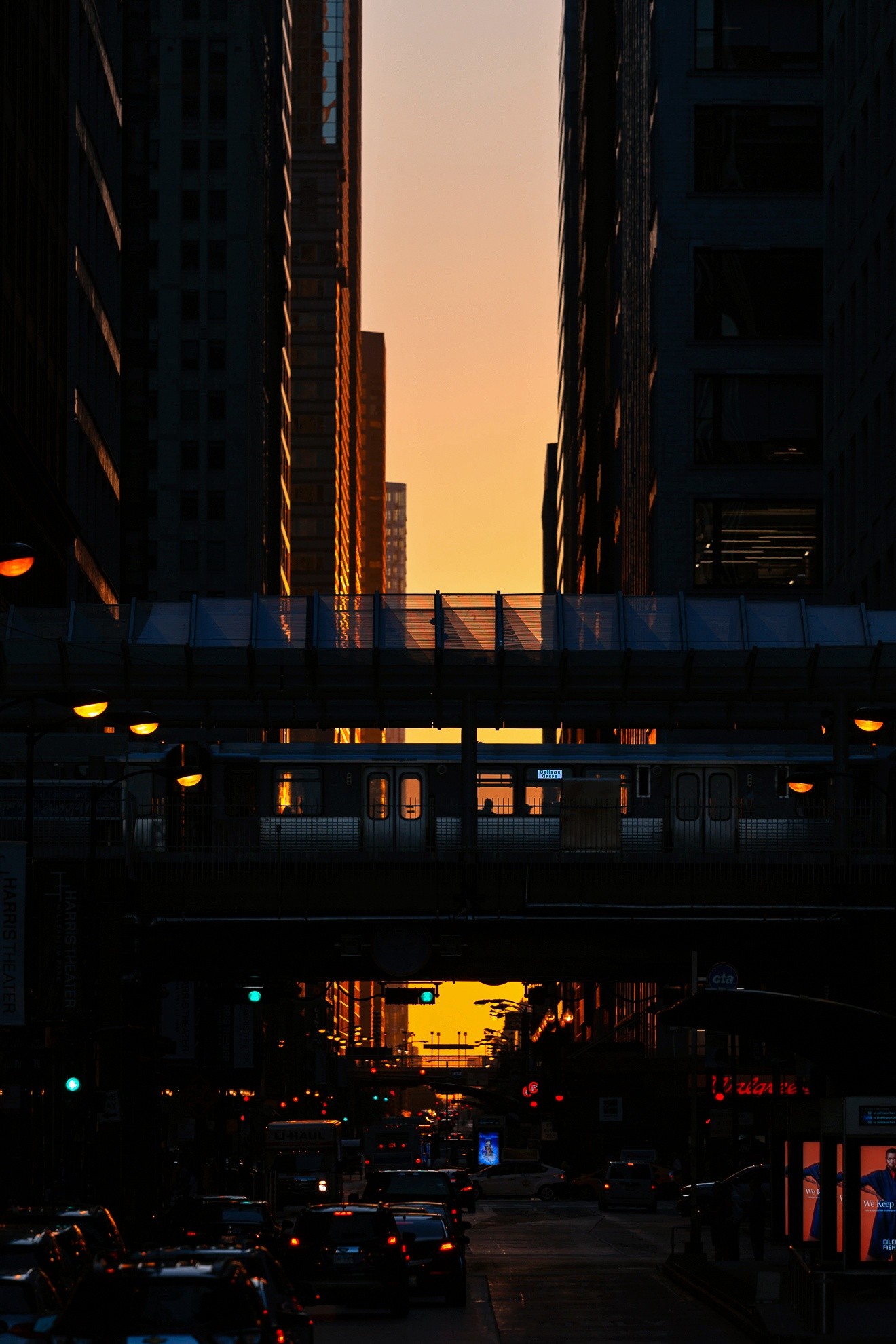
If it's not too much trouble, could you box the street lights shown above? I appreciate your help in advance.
[402,1031,408,1067]
[384,1032,387,1048]
[430,1032,440,1067]
[457,1031,468,1068]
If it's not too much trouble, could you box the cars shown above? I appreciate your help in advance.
[571,1162,681,1203]
[467,1159,565,1201]
[341,1140,361,1174]
[418,1109,461,1152]
[386,1200,471,1310]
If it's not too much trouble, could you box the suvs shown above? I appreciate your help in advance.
[349,1169,476,1213]
[446,1139,474,1167]
[282,1202,414,1315]
[600,1162,658,1206]
[0,1205,127,1344]
[680,1164,777,1226]
[57,1245,321,1344]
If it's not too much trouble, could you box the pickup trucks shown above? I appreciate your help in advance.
[198,1195,270,1235]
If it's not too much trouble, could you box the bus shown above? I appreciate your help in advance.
[86,742,896,859]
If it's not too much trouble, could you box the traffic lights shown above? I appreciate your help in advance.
[385,988,435,1004]
[245,988,292,1004]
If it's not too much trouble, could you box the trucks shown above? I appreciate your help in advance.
[264,1118,342,1212]
[360,1116,424,1170]
